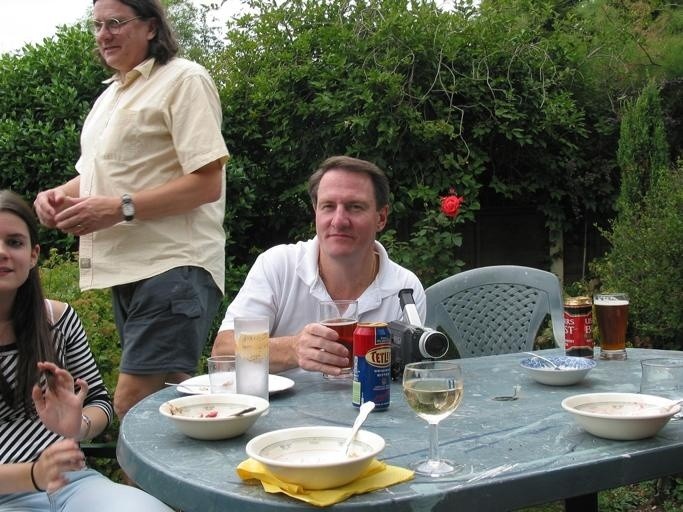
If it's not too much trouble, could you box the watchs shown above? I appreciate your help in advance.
[120,193,135,222]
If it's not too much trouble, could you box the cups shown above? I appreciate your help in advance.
[319,299,359,381]
[640,356,683,422]
[593,292,630,361]
[233,314,270,402]
[207,354,236,393]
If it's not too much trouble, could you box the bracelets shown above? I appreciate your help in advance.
[82,415,91,440]
[31,461,47,493]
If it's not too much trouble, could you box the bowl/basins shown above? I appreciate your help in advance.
[158,393,270,442]
[244,424,387,493]
[519,355,597,386]
[559,392,683,443]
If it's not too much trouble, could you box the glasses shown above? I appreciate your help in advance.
[90,16,142,36]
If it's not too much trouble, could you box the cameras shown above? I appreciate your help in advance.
[387,288,450,382]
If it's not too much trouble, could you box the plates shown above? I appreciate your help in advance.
[176,372,297,396]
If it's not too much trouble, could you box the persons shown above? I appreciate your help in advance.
[33,0,230,424]
[211,155,426,376]
[0,190,177,512]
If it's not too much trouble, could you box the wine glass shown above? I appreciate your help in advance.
[400,359,469,476]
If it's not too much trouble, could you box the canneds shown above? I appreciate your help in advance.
[564,296,593,359]
[352,322,391,411]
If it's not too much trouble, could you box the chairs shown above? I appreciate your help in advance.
[423,265,564,358]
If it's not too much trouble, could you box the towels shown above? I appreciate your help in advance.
[234,458,414,508]
